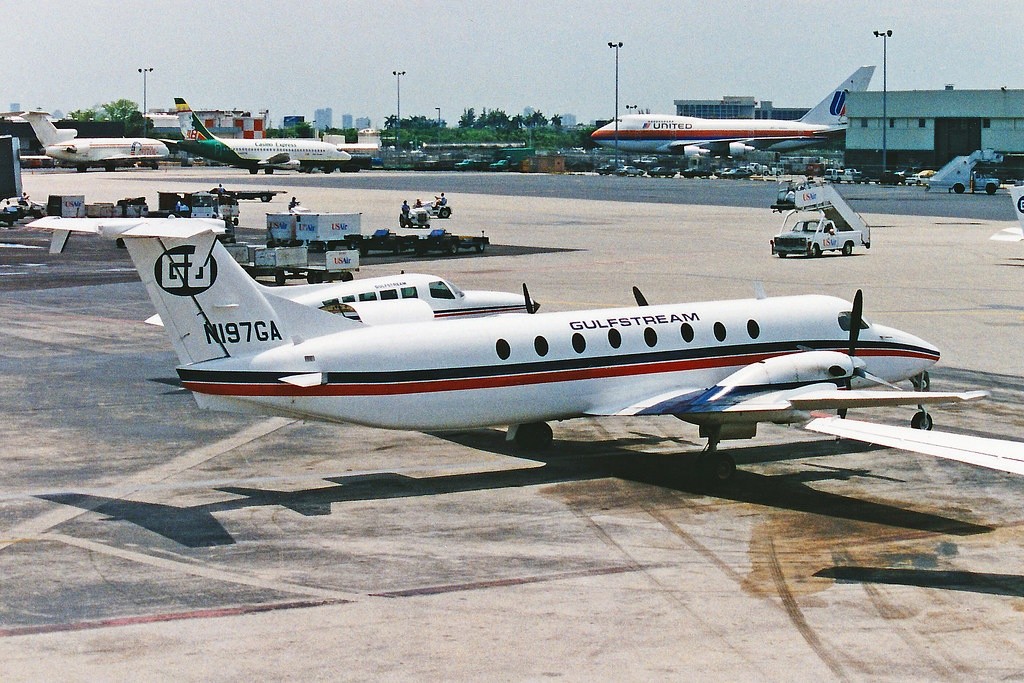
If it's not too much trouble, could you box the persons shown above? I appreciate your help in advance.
[431,192,446,210]
[218,183,226,196]
[289,197,300,210]
[3,201,12,212]
[402,200,410,219]
[18,192,31,206]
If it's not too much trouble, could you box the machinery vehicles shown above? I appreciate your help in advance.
[769,181,870,258]
[928,148,1004,194]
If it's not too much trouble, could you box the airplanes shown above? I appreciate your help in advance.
[19,110,169,171]
[159,97,351,174]
[590,66,876,162]
[104,213,1024,481]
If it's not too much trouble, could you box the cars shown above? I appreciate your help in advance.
[721,169,753,179]
[904,174,930,186]
[615,166,646,176]
[633,157,658,164]
[680,167,713,179]
[647,167,677,177]
[595,164,622,175]
[714,168,733,178]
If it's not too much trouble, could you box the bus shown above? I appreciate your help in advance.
[764,162,785,176]
[852,166,884,184]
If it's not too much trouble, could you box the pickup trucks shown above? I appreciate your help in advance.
[454,159,489,171]
[489,159,523,171]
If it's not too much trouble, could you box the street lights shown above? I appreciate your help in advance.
[393,71,407,156]
[873,29,892,173]
[435,108,441,154]
[625,105,637,114]
[138,68,154,138]
[607,42,623,166]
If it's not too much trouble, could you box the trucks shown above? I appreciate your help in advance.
[823,169,861,183]
[191,192,240,218]
